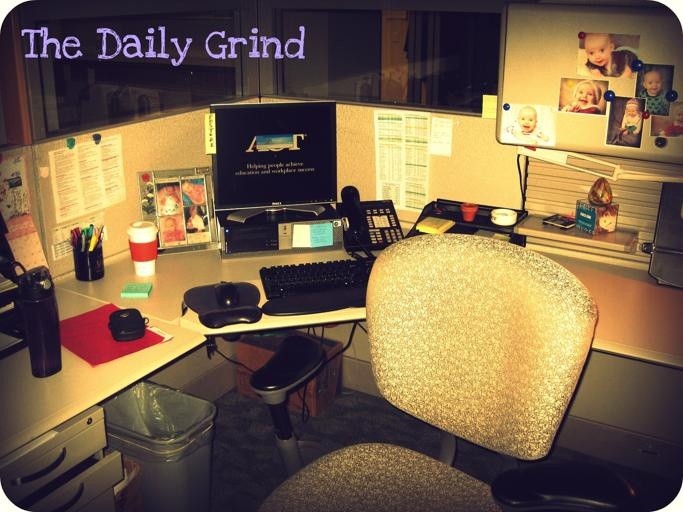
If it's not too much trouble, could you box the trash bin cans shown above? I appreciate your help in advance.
[98,380,217,512]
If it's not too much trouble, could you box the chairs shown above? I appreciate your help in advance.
[257,232,607,512]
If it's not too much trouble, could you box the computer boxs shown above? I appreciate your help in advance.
[215,204,344,258]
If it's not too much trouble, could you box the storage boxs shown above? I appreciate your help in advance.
[234,331,347,419]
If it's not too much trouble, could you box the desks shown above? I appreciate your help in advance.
[1,90,683,511]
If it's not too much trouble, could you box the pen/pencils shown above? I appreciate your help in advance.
[70,223,105,252]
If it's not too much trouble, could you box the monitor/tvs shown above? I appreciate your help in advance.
[209,101,337,224]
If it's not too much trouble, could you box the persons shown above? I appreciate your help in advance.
[504,106,549,149]
[636,69,669,117]
[657,101,683,137]
[617,99,644,138]
[579,32,638,80]
[560,80,605,115]
[157,179,207,244]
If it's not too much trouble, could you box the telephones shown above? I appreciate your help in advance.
[336,186,405,252]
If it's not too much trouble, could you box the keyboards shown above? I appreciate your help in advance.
[259,257,379,300]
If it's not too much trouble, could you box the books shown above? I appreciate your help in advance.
[513,210,640,252]
[0,212,50,362]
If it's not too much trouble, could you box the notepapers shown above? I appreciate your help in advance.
[121,282,152,298]
[416,216,456,234]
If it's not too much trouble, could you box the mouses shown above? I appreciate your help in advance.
[214,280,240,308]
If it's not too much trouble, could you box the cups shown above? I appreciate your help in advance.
[73,246,106,283]
[461,202,479,223]
[126,219,158,276]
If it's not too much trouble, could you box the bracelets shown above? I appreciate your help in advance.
[537,132,542,138]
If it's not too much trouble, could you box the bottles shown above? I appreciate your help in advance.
[18,265,63,379]
